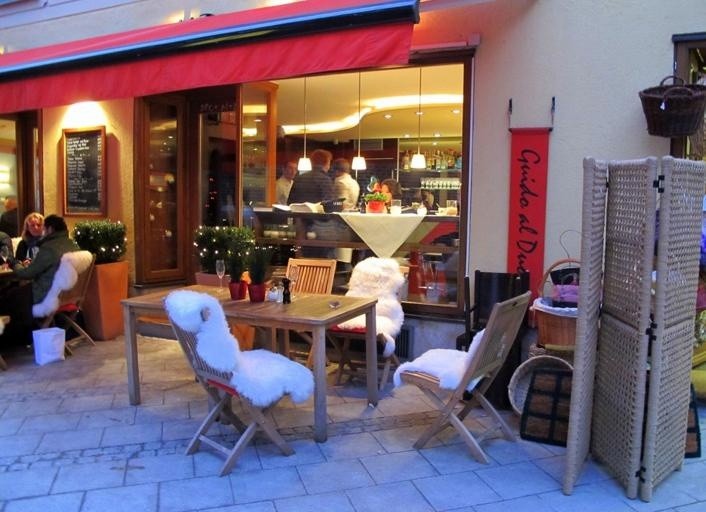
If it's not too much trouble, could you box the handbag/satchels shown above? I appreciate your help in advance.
[32,327,66,365]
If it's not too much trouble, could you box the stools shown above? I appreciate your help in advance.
[418,251,445,294]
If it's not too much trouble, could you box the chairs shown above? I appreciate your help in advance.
[278,254,340,373]
[164,290,313,478]
[391,294,531,466]
[457,268,532,412]
[26,250,98,354]
[325,254,411,390]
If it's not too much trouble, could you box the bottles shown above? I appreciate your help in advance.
[404,149,462,170]
[360,196,367,213]
[267,286,284,303]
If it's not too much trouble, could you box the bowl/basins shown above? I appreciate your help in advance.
[365,200,386,213]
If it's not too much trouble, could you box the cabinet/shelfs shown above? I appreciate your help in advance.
[396,140,464,211]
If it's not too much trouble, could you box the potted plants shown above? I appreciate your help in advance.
[258,222,317,240]
[361,193,386,213]
[243,248,274,303]
[190,222,257,288]
[70,217,132,343]
[227,250,247,301]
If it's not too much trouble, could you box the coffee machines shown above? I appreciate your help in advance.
[421,231,458,303]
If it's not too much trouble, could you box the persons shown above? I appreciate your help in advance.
[378,178,437,213]
[275,159,298,203]
[13,211,46,268]
[0,213,82,365]
[331,157,362,209]
[285,148,347,213]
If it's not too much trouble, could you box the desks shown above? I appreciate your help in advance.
[242,210,459,300]
[116,282,380,443]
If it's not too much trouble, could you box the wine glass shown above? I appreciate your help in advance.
[0,244,10,271]
[216,258,226,291]
[288,263,299,302]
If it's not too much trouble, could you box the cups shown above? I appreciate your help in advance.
[438,206,447,214]
[419,176,462,190]
[447,200,457,216]
[390,198,402,215]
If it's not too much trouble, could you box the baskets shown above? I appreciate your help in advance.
[533,258,580,348]
[639,76,706,136]
[509,356,574,415]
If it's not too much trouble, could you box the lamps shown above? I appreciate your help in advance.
[408,68,427,171]
[350,73,367,174]
[295,78,316,173]
